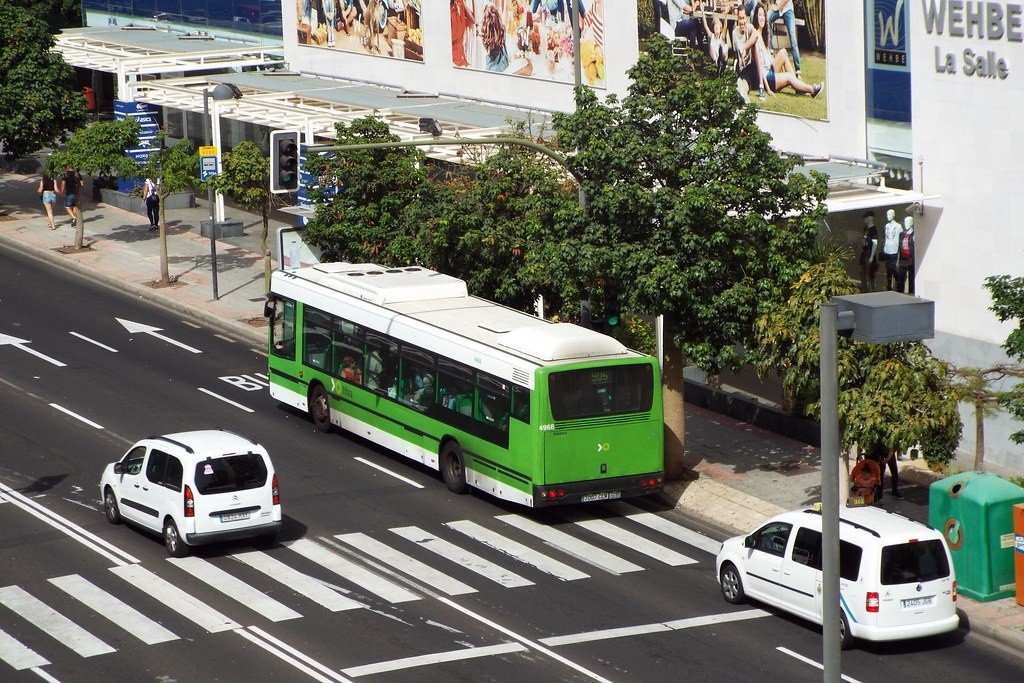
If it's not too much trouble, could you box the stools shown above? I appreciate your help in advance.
[671,37,689,56]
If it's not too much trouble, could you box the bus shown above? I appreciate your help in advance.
[263,261,665,509]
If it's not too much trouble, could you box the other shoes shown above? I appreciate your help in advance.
[718,46,724,74]
[759,88,764,101]
[795,73,802,80]
[879,492,883,500]
[71,217,76,227]
[811,82,824,99]
[149,224,155,231]
[890,491,904,499]
[154,225,158,231]
[48,224,56,230]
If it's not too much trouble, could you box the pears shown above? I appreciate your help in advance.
[407,28,423,45]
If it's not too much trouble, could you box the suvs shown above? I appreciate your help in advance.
[715,505,970,651]
[99,427,282,558]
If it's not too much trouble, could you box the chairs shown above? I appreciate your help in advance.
[339,362,494,422]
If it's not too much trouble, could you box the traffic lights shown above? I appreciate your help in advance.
[270,130,300,193]
[604,283,621,328]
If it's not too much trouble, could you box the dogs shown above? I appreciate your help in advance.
[364,0,382,55]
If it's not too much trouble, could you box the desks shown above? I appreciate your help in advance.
[694,11,805,54]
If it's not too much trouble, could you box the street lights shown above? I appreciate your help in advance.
[820,291,934,683]
[204,85,233,300]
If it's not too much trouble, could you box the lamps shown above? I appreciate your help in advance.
[889,166,911,181]
[418,117,443,137]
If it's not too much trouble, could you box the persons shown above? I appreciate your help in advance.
[881,209,903,291]
[61,164,84,226]
[854,464,877,498]
[450,0,604,73]
[275,339,435,408]
[859,210,878,293]
[143,176,160,230]
[867,438,905,500]
[322,0,357,48]
[895,215,915,297]
[667,0,824,103]
[37,170,59,230]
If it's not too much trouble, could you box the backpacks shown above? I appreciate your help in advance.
[148,192,160,207]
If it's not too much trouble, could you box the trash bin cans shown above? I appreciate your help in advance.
[929,470,1024,603]
